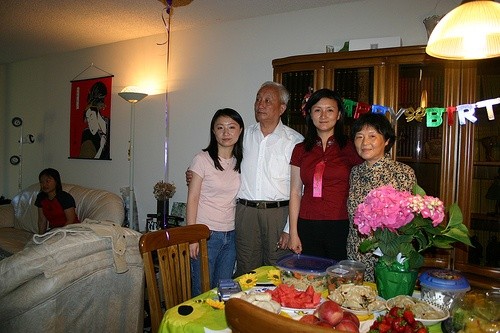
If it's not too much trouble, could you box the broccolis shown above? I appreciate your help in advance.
[451,307,492,333]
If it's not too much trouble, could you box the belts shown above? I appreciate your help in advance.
[237,198,289,209]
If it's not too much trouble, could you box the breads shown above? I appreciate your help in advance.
[241,293,280,314]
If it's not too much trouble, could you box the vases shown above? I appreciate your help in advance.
[373,263,418,300]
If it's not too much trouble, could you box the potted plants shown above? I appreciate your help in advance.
[152,180,177,230]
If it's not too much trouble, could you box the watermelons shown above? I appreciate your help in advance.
[265,283,322,309]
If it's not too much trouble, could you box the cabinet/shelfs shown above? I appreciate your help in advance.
[272,45,500,291]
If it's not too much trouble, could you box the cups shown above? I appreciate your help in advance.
[326,265,356,293]
[338,260,366,286]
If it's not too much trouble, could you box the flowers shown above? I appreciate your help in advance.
[354,182,475,273]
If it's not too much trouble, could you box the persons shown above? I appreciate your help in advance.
[186,81,306,278]
[346,110,417,283]
[34,168,80,234]
[288,88,362,261]
[187,108,245,298]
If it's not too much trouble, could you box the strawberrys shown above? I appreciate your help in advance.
[370,304,429,333]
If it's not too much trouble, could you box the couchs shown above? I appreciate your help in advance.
[0,228,144,333]
[0,182,125,261]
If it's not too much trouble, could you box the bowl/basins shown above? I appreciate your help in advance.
[419,270,470,301]
[444,290,500,333]
[385,296,449,325]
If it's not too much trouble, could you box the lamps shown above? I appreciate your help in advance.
[118,87,149,230]
[9,113,34,194]
[425,0,500,60]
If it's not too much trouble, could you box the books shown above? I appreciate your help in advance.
[283,68,500,271]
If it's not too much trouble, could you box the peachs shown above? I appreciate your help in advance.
[299,301,360,333]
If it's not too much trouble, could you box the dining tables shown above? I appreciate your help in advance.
[159,266,441,333]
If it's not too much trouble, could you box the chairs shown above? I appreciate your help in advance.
[139,224,210,333]
[145,213,184,233]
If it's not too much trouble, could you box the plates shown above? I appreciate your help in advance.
[251,287,326,311]
[327,293,388,313]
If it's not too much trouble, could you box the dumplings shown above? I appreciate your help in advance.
[327,283,385,311]
[390,295,441,319]
